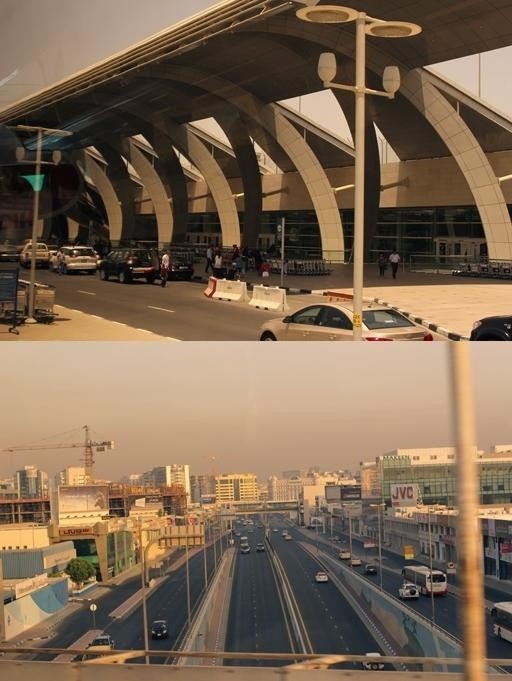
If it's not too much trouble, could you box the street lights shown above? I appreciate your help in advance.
[287,2,421,340]
[412,502,444,624]
[344,502,356,548]
[326,502,339,538]
[15,121,73,325]
[370,504,385,591]
[141,534,204,664]
[187,504,235,625]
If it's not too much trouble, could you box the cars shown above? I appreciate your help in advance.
[350,557,362,567]
[334,536,346,544]
[338,550,352,561]
[466,309,512,340]
[233,513,292,556]
[156,254,195,281]
[365,564,377,575]
[2,238,102,277]
[253,300,435,340]
[314,569,328,584]
[88,636,116,649]
[151,618,170,639]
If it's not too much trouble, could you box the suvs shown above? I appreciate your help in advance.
[399,584,419,601]
[360,651,385,670]
[100,248,158,283]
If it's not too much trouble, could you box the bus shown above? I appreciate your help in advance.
[399,565,448,597]
[489,600,512,645]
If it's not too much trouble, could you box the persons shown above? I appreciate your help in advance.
[377,252,386,277]
[204,244,272,283]
[55,247,64,273]
[388,249,401,280]
[158,247,170,288]
[283,255,289,278]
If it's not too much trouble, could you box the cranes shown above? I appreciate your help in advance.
[1,420,119,476]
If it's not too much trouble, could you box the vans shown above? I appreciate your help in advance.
[81,645,113,664]
